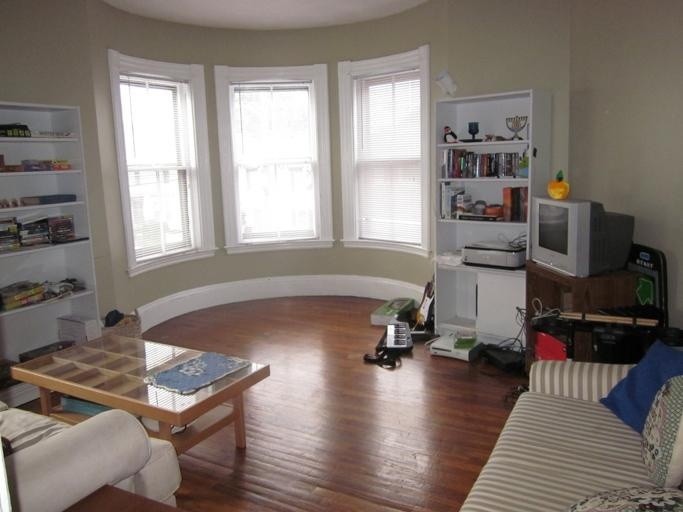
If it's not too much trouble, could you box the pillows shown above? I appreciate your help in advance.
[569,334,681,510]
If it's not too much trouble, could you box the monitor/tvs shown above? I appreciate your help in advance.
[531,195,635,279]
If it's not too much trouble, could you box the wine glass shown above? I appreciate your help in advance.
[468,121,479,140]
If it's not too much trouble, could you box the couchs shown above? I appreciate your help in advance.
[459,360,656,509]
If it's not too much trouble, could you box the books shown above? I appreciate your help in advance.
[0,278,76,313]
[20,193,76,205]
[0,212,76,251]
[437,147,529,222]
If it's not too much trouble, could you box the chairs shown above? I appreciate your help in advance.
[1,404,182,509]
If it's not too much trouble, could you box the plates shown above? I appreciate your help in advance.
[459,139,483,142]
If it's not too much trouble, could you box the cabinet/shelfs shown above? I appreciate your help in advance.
[431,89,552,353]
[525,264,635,381]
[0,102,105,410]
